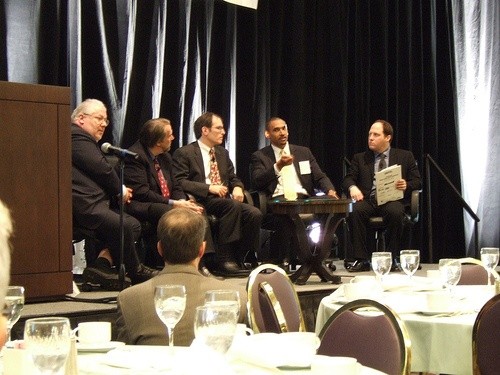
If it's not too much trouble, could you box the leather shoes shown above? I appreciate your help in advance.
[348,259,371,272]
[390,257,398,272]
[200,256,290,281]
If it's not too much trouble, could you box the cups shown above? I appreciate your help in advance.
[195,289,241,361]
[73,321,111,345]
[439,258,461,290]
[24,317,71,375]
[400,250,421,277]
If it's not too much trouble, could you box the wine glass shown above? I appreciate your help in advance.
[155,285,187,349]
[481,248,499,289]
[372,251,392,294]
[1,286,26,346]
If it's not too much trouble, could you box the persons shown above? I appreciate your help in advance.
[69,98,164,290]
[250,117,338,271]
[0,199,13,357]
[172,112,264,278]
[110,207,248,347]
[109,118,225,280]
[344,121,425,272]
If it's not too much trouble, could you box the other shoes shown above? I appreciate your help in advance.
[126,263,161,285]
[82,263,132,291]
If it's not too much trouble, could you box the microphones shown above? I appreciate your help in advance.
[100,142,138,160]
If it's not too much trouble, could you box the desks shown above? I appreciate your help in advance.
[0,340,387,375]
[274,198,349,285]
[316,263,500,375]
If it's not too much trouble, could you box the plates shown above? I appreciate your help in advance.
[77,342,125,351]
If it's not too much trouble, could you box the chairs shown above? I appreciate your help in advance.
[343,189,423,269]
[186,190,267,219]
[472,294,500,375]
[246,263,306,332]
[317,298,410,375]
[258,282,288,333]
[448,257,500,285]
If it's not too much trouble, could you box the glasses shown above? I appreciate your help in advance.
[83,113,110,127]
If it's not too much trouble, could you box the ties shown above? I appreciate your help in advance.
[378,154,387,171]
[280,149,298,200]
[152,158,171,199]
[209,149,222,186]
